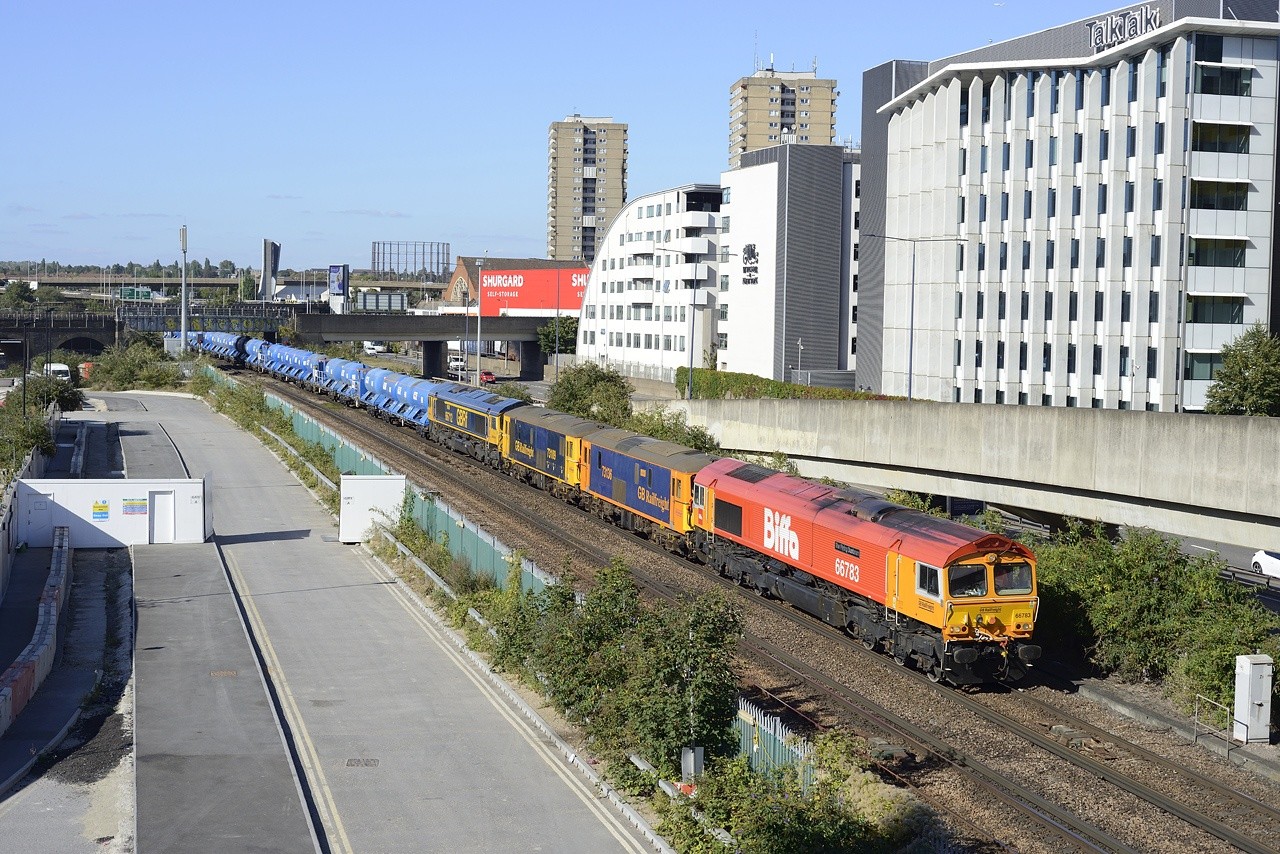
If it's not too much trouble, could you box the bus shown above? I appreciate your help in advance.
[363,340,387,353]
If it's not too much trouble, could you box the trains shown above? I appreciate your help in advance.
[163,329,1043,691]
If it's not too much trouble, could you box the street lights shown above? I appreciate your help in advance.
[528,257,583,388]
[861,233,969,401]
[27,261,317,315]
[475,258,484,388]
[655,247,738,399]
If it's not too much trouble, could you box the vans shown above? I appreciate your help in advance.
[43,363,71,385]
[447,355,464,371]
[364,346,377,358]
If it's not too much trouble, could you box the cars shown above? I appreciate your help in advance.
[1251,549,1280,580]
[480,369,496,385]
